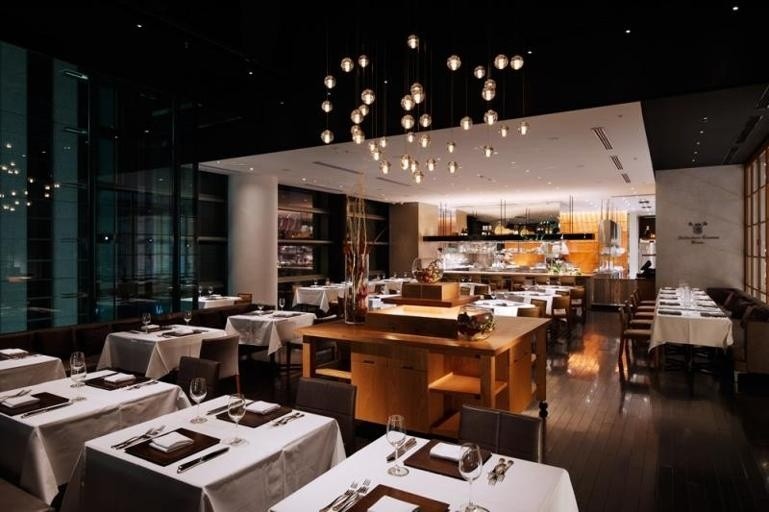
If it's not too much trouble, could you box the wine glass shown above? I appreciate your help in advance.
[142,313,152,337]
[387,414,410,476]
[190,377,207,424]
[279,298,285,313]
[70,352,87,401]
[458,443,481,511]
[227,393,247,446]
[184,310,192,325]
[198,285,213,299]
[257,299,264,319]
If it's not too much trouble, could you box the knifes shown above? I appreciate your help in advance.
[386,437,417,463]
[177,446,230,474]
[206,400,244,416]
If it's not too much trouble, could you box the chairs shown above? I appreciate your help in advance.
[617,286,769,371]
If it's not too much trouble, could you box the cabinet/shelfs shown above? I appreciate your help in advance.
[347,196,390,277]
[278,185,349,293]
[117,166,228,292]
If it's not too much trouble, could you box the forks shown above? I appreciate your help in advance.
[488,460,513,486]
[110,425,166,450]
[319,478,372,512]
[271,413,305,425]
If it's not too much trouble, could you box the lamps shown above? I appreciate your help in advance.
[321,98,332,113]
[495,122,509,138]
[481,87,496,101]
[406,34,420,48]
[401,114,415,129]
[352,129,365,144]
[361,78,375,105]
[359,103,369,116]
[494,51,508,70]
[446,139,456,153]
[407,131,415,143]
[399,151,412,172]
[350,105,364,124]
[516,117,527,137]
[378,160,392,177]
[420,112,432,128]
[418,132,432,150]
[425,154,436,172]
[379,134,388,149]
[340,47,353,72]
[447,54,461,71]
[460,114,473,130]
[318,0,527,185]
[411,82,423,95]
[351,125,360,134]
[372,148,381,161]
[510,51,524,71]
[447,159,458,175]
[483,109,498,126]
[320,121,334,144]
[324,71,336,89]
[482,141,494,158]
[367,138,377,153]
[473,64,486,79]
[409,158,419,173]
[484,76,496,90]
[414,167,424,184]
[358,43,369,68]
[413,92,425,104]
[401,94,415,111]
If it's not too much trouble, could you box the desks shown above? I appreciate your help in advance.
[0,344,585,512]
[94,269,587,434]
